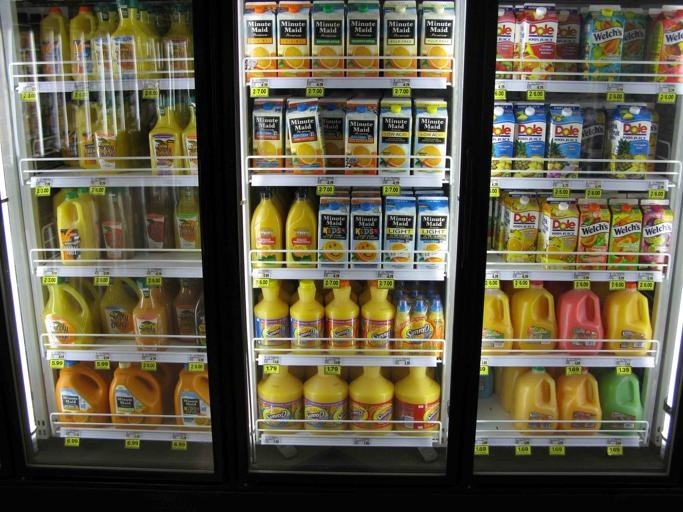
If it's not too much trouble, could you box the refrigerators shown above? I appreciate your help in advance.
[0,1,682,511]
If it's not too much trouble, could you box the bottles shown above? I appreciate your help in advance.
[131,278,207,352]
[99,184,201,258]
[57,92,197,175]
[18,1,194,80]
[251,190,316,268]
[257,366,440,436]
[254,279,444,357]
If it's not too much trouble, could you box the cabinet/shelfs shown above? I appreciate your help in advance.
[461,0,681,512]
[0,1,235,511]
[215,0,477,512]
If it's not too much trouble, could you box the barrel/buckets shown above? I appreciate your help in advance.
[42,277,90,350]
[101,277,139,340]
[68,6,95,78]
[482,280,656,436]
[48,362,211,429]
[55,192,91,263]
[256,359,443,435]
[35,5,71,83]
